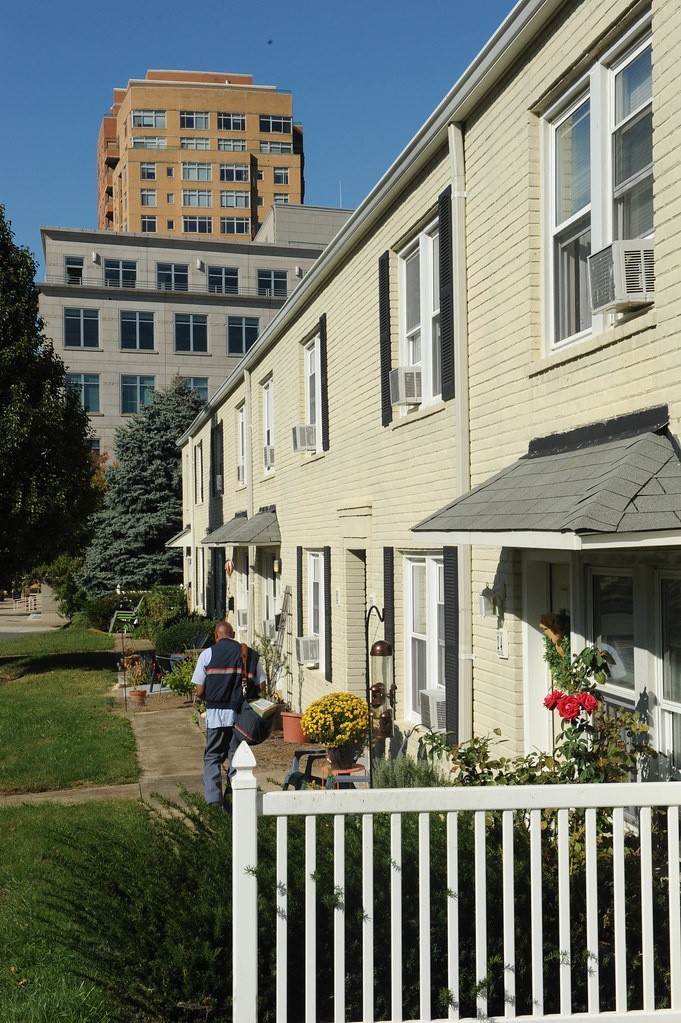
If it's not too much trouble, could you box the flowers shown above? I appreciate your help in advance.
[300,693,379,749]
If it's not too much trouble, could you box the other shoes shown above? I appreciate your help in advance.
[209,802,222,821]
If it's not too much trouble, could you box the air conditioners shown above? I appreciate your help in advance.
[419,690,448,735]
[389,366,422,406]
[295,636,321,665]
[586,239,655,316]
[479,596,494,616]
[263,619,275,639]
[217,445,274,491]
[292,424,318,452]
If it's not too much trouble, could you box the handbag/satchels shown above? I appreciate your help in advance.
[232,698,275,745]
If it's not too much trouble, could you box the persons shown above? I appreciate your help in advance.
[191,622,268,817]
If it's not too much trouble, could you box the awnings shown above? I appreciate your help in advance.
[408,406,681,555]
[200,505,281,546]
[164,524,192,550]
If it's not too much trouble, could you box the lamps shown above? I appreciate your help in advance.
[274,559,281,573]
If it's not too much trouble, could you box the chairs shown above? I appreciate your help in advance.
[282,724,371,791]
[108,595,146,634]
[149,633,213,696]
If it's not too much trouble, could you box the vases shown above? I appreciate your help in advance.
[280,710,313,745]
[325,742,358,771]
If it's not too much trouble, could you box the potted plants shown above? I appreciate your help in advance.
[124,654,148,707]
[249,629,296,732]
